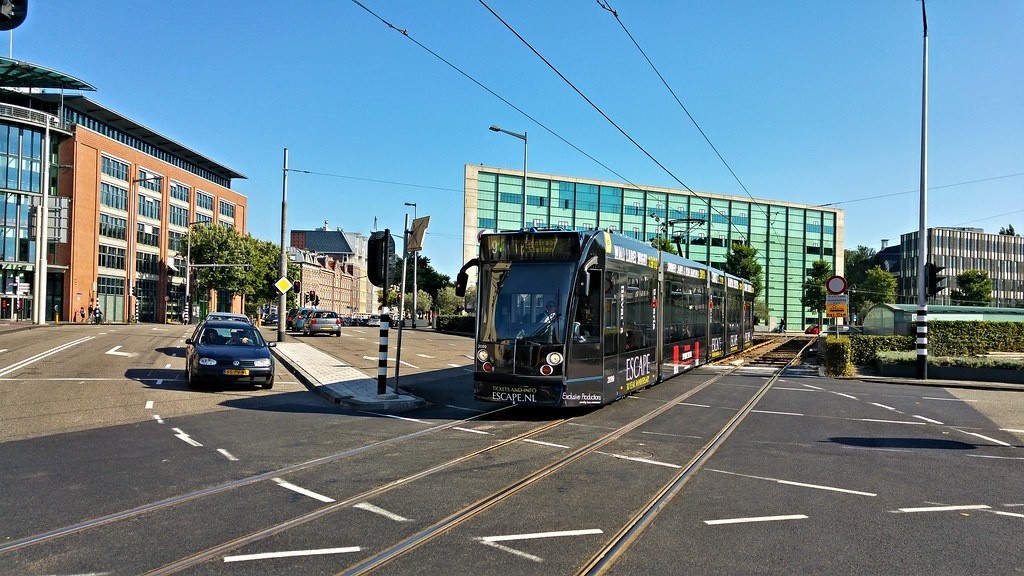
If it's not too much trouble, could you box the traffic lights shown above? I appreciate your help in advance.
[309,291,316,302]
[316,295,320,305]
[293,280,301,294]
[925,263,947,297]
[306,295,309,303]
[397,294,401,305]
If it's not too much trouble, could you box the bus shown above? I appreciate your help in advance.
[455,219,755,407]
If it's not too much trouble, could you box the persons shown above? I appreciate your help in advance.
[88,304,103,324]
[80,307,86,323]
[537,301,565,339]
[225,331,253,345]
[204,331,223,345]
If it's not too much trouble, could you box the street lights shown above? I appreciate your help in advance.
[404,201,417,328]
[184,220,210,324]
[489,125,527,229]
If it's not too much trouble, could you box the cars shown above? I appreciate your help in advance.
[286,308,298,330]
[301,310,342,337]
[389,317,399,327]
[805,324,822,334]
[185,319,277,390]
[293,308,317,332]
[339,315,381,326]
[264,314,279,326]
[206,312,251,337]
